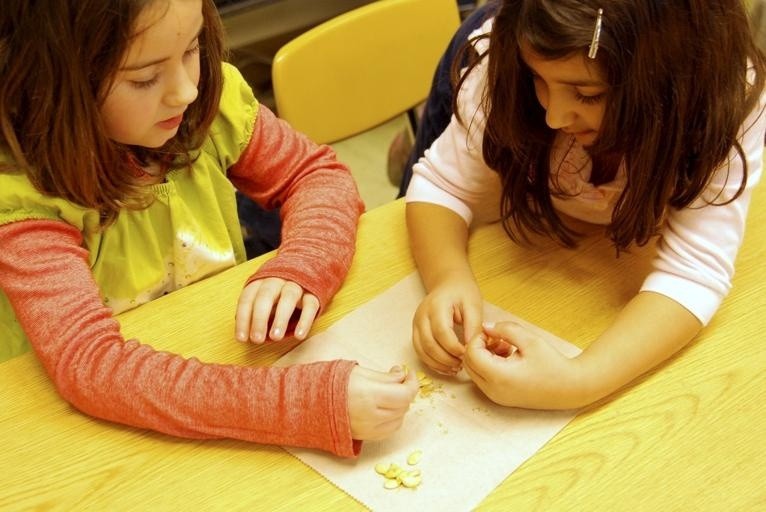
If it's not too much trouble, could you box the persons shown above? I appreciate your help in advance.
[0,0,418,462]
[403,0,766,413]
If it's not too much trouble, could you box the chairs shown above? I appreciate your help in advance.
[271,1,464,221]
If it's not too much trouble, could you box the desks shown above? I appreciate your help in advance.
[0,142,766,512]
